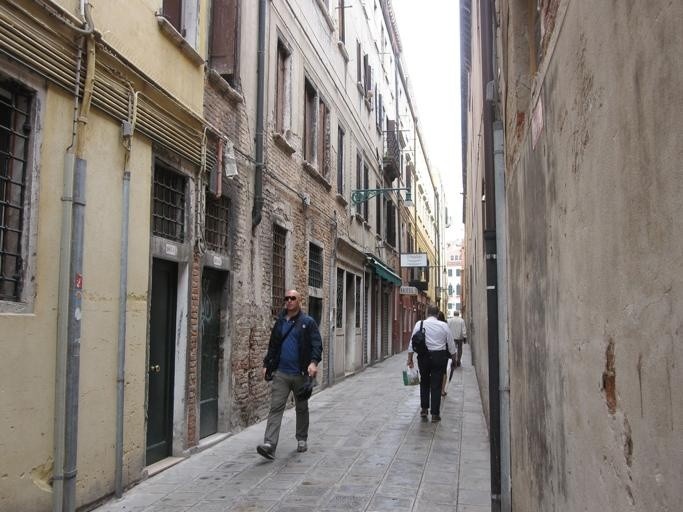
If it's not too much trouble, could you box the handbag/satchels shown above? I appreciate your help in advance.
[402,364,420,386]
[262,348,281,369]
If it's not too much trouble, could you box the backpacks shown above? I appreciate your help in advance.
[411,320,429,355]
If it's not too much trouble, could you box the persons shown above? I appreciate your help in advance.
[437,311,457,396]
[256,290,323,459]
[446,311,467,367]
[407,305,457,422]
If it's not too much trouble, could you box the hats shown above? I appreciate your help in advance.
[295,376,317,403]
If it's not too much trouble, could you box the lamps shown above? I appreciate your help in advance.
[349,187,413,209]
[418,264,447,275]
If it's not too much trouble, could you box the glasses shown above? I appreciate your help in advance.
[283,296,297,302]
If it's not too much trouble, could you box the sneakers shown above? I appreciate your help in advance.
[420,408,428,418]
[431,415,441,422]
[297,439,308,453]
[256,442,276,460]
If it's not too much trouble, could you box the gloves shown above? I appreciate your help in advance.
[265,368,275,383]
